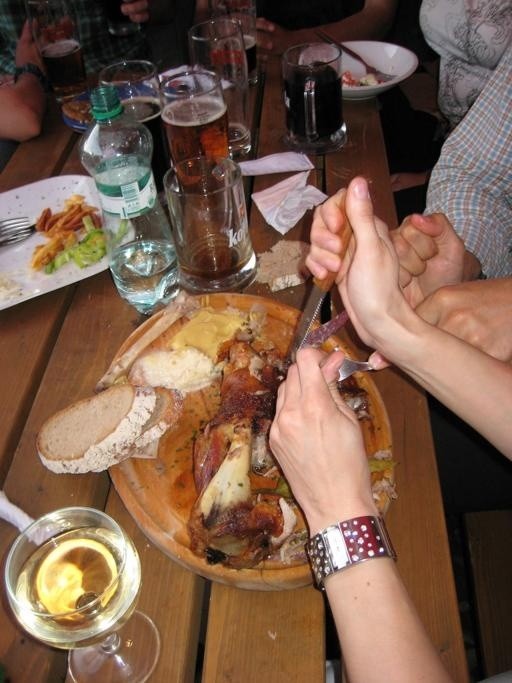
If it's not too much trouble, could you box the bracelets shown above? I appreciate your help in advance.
[303,516,400,592]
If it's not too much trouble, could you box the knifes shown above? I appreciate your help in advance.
[286,210,354,366]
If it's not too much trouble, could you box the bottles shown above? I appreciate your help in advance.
[81,86,183,315]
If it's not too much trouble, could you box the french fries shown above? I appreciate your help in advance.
[35,203,102,237]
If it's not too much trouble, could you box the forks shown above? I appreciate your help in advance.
[0,217,29,244]
[313,30,397,83]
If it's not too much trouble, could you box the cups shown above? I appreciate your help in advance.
[159,71,231,219]
[187,18,253,156]
[282,43,349,156]
[106,0,141,36]
[212,0,257,87]
[164,156,258,292]
[25,0,89,102]
[99,60,173,207]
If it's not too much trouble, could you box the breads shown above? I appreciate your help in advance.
[35,383,184,475]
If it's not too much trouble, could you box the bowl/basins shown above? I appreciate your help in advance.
[298,40,419,100]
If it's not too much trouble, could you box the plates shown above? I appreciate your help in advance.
[0,174,135,313]
[143,65,233,104]
[105,292,393,591]
[63,83,169,130]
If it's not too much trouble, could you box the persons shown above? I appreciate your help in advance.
[267,175,510,683]
[2,2,511,191]
[367,26,512,371]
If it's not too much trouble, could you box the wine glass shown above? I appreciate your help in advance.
[5,505,160,683]
